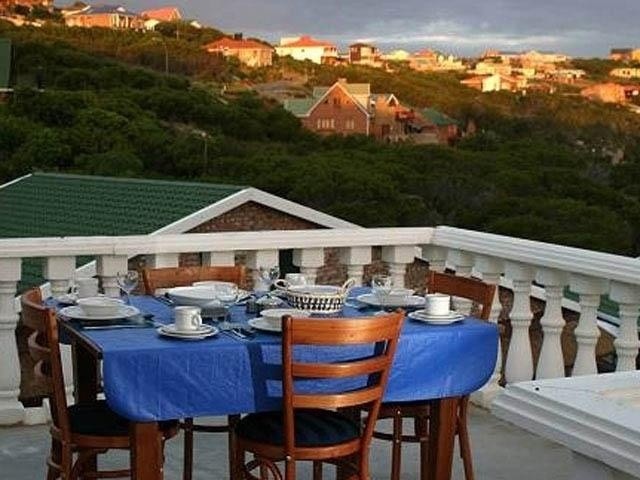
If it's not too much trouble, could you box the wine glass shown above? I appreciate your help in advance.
[371,275,391,318]
[117,270,140,307]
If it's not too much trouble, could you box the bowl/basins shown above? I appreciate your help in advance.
[77,297,127,317]
[166,278,240,318]
[373,289,416,303]
[59,302,142,321]
[259,307,312,327]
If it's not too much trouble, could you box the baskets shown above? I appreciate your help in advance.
[273,277,356,314]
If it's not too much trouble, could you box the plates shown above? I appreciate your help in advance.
[156,321,218,342]
[407,308,466,325]
[355,293,426,312]
[414,310,461,319]
[57,293,107,305]
[162,324,214,335]
[248,316,309,332]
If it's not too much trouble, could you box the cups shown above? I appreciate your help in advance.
[285,272,309,286]
[424,293,452,317]
[71,277,99,299]
[174,305,202,330]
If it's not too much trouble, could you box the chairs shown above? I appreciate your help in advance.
[359,269,497,479]
[21,288,180,480]
[142,265,247,480]
[229,308,407,478]
